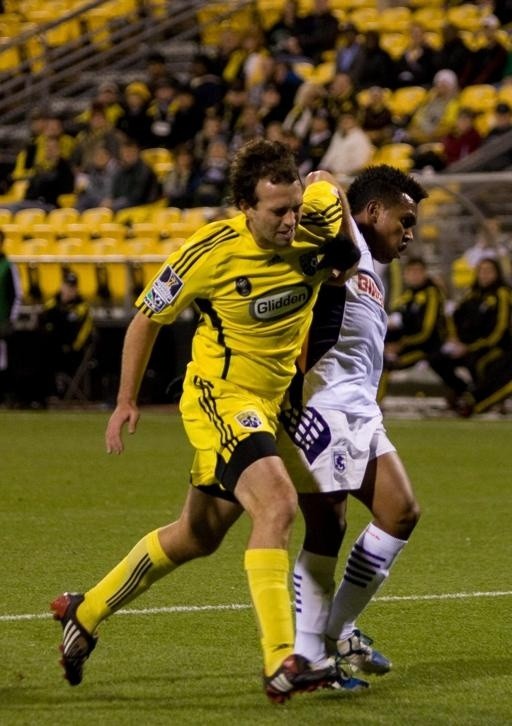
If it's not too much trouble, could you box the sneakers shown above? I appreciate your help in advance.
[49,592,100,686]
[263,627,392,705]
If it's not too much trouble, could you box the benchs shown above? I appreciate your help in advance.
[1,207,235,304]
[199,1,509,61]
[2,2,165,73]
[356,84,512,137]
